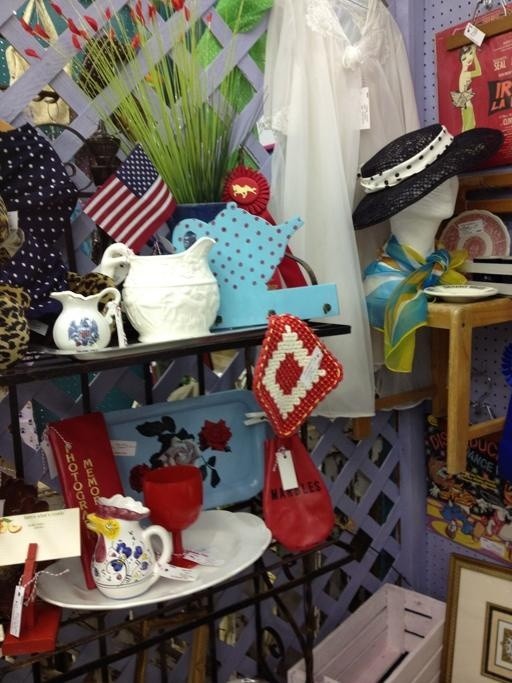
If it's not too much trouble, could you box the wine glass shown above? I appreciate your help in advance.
[143,465,204,567]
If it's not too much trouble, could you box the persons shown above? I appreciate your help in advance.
[449,42,482,133]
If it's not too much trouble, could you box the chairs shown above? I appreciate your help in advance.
[423,174,512,476]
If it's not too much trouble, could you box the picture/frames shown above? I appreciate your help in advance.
[440,555,512,683]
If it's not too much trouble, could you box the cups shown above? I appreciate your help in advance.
[49,287,123,351]
[86,513,172,602]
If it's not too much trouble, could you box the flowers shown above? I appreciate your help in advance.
[23,2,270,206]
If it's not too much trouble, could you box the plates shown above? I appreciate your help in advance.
[440,209,510,262]
[424,285,498,302]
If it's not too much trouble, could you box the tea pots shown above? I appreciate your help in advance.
[171,201,305,284]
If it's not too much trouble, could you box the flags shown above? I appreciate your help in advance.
[82,144,177,256]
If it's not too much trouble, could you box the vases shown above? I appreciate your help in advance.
[175,206,217,232]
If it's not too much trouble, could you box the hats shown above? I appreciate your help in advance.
[352,123,504,230]
